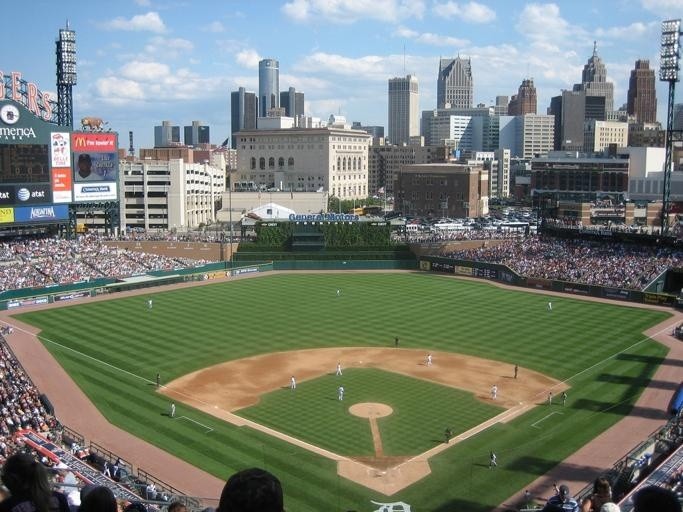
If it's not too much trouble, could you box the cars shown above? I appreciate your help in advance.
[384,207,543,232]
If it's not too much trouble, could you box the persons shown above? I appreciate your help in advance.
[337,385,345,401]
[393,335,400,349]
[547,300,553,312]
[336,362,343,377]
[560,391,568,405]
[148,298,153,311]
[524,489,531,501]
[672,323,682,341]
[542,414,682,511]
[489,384,498,401]
[290,374,296,389]
[489,450,499,470]
[513,364,519,378]
[444,427,453,444]
[427,354,432,367]
[0,225,255,294]
[335,288,340,297]
[0,324,187,511]
[547,392,553,406]
[218,466,284,511]
[393,198,682,290]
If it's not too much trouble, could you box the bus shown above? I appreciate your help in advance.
[354,207,379,217]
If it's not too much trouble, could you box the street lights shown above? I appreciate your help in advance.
[660,18,683,239]
[54,18,77,128]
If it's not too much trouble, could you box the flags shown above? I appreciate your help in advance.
[212,136,229,153]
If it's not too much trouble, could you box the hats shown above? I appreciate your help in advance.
[80,484,117,512]
[560,485,569,497]
